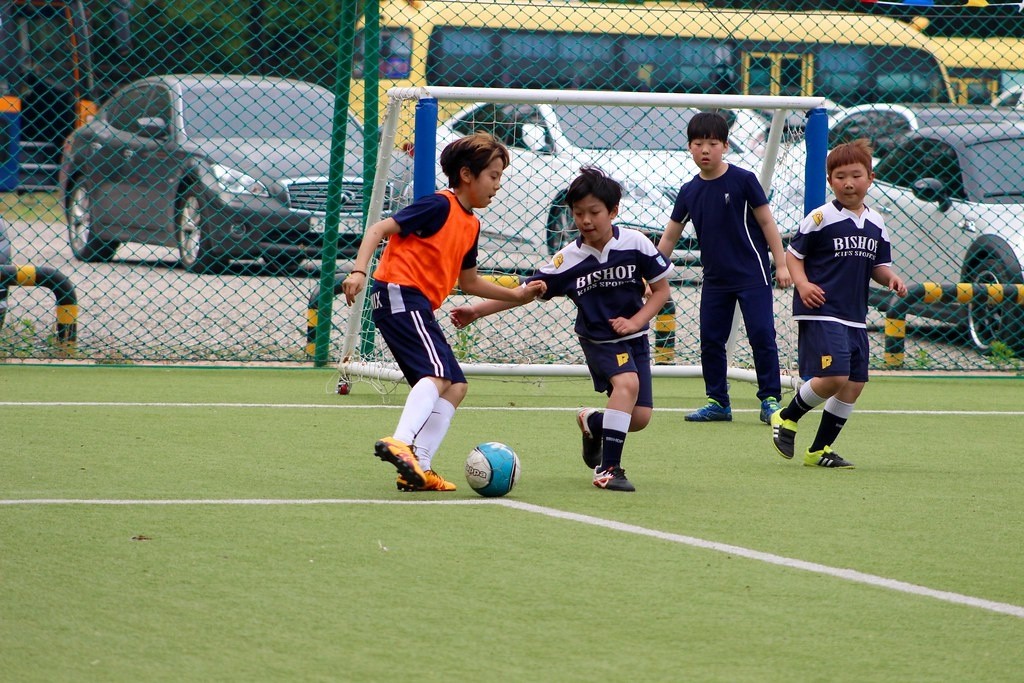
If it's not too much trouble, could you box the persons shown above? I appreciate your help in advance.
[449,165,675,492]
[340,130,547,491]
[657,113,793,426]
[771,138,908,470]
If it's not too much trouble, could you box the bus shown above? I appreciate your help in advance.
[349,0,959,158]
[922,31,1024,111]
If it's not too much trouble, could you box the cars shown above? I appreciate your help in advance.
[429,99,801,285]
[57,74,415,274]
[794,99,1024,359]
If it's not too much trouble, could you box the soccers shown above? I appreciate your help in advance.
[464,442,519,497]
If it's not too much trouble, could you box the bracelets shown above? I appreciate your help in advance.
[349,271,366,276]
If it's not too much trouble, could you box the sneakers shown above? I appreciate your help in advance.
[770,407,797,459]
[576,408,603,469]
[375,437,427,488]
[759,397,781,425]
[803,445,854,469]
[593,465,635,492]
[397,466,456,491]
[685,399,732,422]
